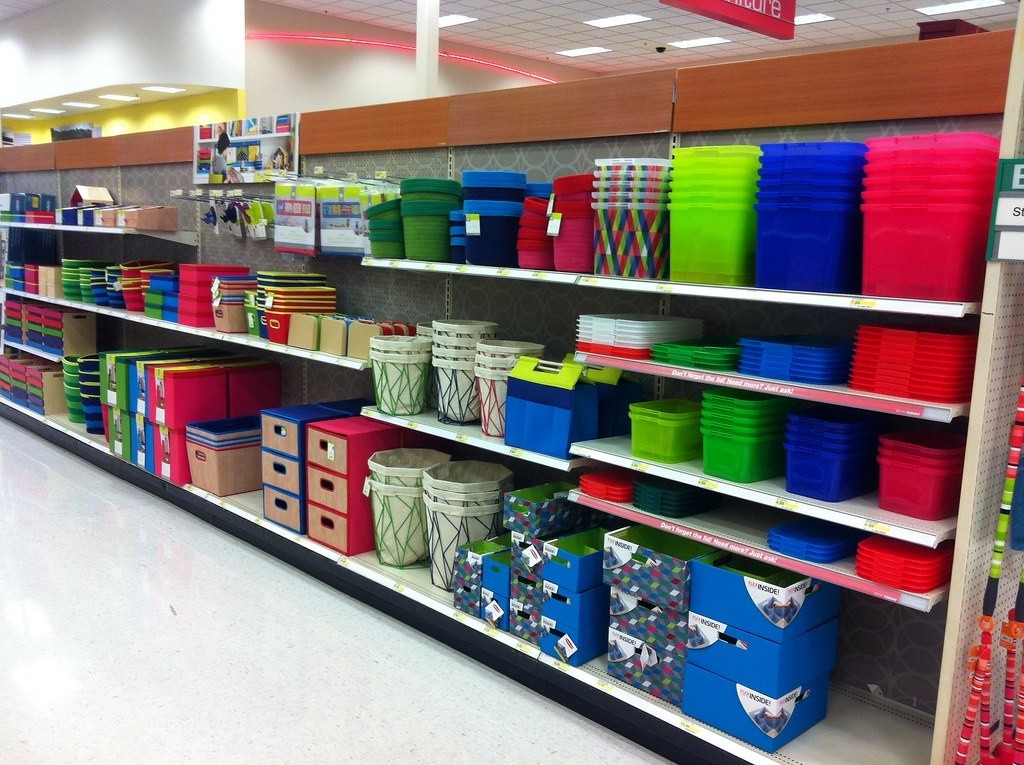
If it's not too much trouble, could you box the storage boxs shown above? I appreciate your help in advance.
[0,131,1005,755]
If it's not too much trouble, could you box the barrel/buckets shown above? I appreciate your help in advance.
[63,353,105,435]
[366,449,515,594]
[369,318,545,438]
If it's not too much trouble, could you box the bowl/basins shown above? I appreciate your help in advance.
[60,258,176,311]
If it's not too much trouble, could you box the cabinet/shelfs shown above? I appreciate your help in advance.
[538,260,1024,765]
[0,220,199,499]
[335,254,603,694]
[165,320,371,588]
